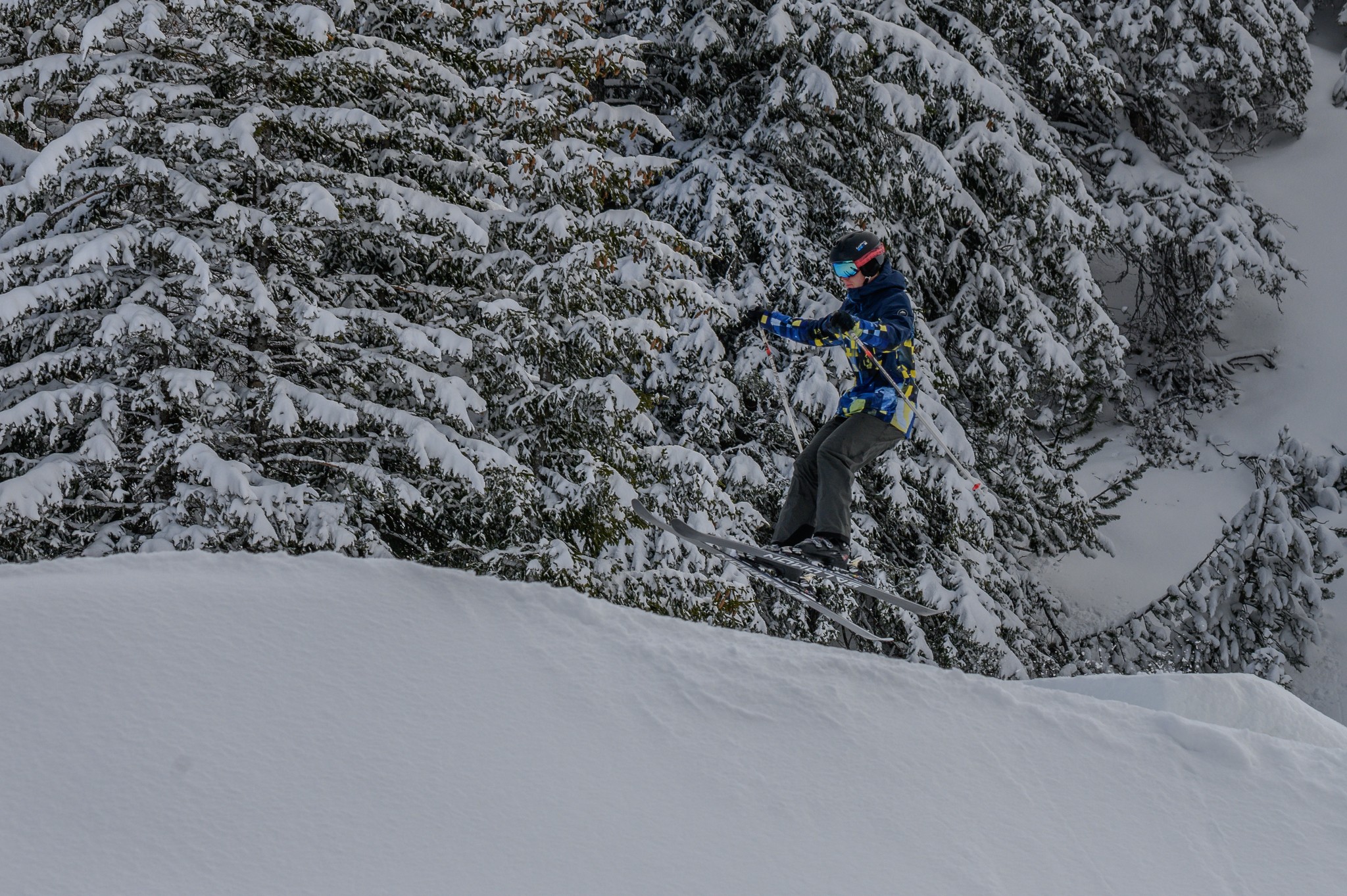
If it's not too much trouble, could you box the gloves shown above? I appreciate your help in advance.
[747,306,766,326]
[830,310,855,334]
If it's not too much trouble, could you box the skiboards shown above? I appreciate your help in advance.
[627,497,955,645]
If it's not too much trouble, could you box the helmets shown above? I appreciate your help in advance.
[828,231,885,278]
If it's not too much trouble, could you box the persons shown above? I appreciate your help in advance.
[749,231,918,591]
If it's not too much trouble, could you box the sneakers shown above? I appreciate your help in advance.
[752,545,814,586]
[794,536,850,572]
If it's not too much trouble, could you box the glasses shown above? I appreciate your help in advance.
[831,260,859,278]
